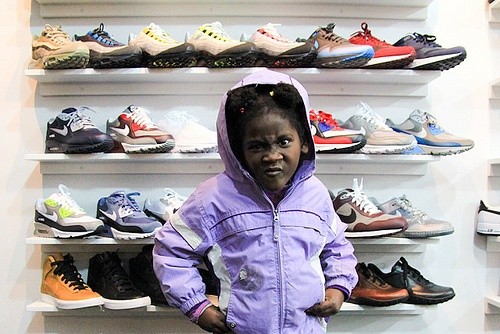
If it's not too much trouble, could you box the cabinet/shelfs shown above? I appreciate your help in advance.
[25,0,440,316]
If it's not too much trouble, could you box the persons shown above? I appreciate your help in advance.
[153,69,359,334]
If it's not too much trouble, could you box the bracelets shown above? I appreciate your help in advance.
[189,300,212,324]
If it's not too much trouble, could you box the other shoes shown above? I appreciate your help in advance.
[386,255,456,306]
[347,260,409,305]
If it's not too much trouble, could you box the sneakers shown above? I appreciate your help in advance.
[130,244,171,308]
[97,104,175,152]
[476,199,500,237]
[96,189,163,241]
[44,107,115,152]
[386,108,474,155]
[30,22,468,70]
[157,109,220,154]
[34,183,106,239]
[38,251,103,310]
[144,188,188,225]
[329,176,408,238]
[376,193,455,237]
[342,102,406,149]
[87,247,151,309]
[306,99,365,153]
